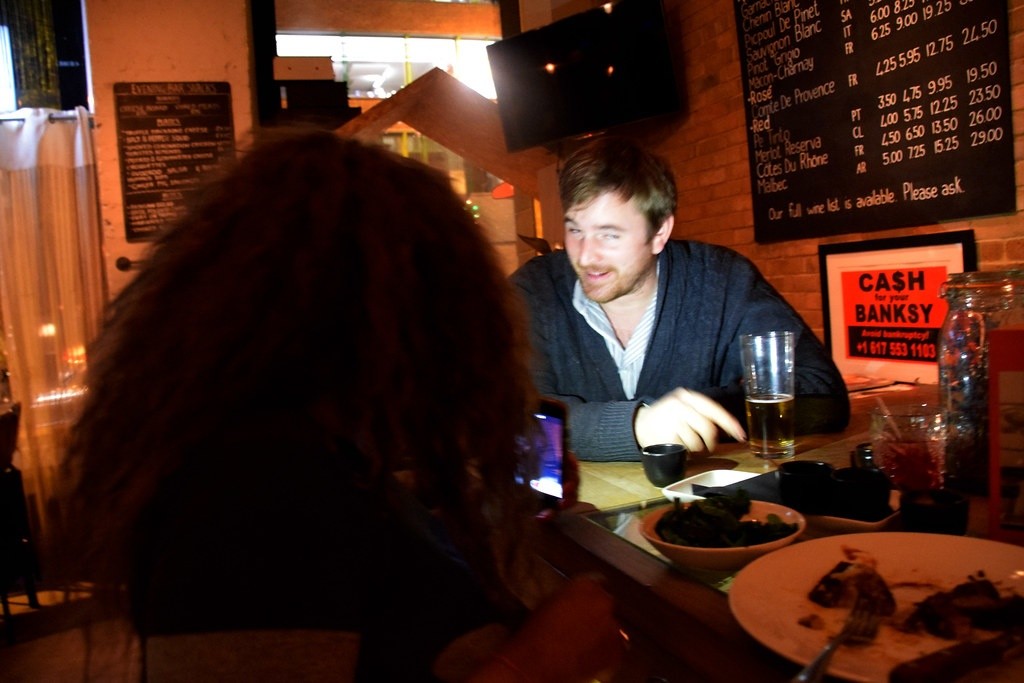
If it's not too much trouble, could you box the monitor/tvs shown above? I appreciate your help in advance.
[488,0,685,153]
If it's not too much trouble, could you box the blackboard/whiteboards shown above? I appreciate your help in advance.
[733,0,1018,244]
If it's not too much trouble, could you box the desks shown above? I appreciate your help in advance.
[533,392,1024,683]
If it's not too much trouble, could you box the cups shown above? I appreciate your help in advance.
[871,404,947,493]
[739,330,796,457]
[641,442,689,488]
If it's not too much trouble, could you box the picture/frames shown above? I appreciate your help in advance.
[818,228,978,381]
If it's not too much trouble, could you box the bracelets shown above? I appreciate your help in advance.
[496,656,530,683]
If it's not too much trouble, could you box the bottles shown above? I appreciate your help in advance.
[937,273,1015,480]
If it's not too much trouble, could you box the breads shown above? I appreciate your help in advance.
[810,559,1024,641]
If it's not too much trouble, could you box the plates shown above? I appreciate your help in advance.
[780,477,907,535]
[729,533,1015,683]
[661,470,762,504]
[640,497,807,573]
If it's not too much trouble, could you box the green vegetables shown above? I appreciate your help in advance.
[657,486,798,548]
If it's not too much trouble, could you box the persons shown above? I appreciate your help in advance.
[508,138,852,464]
[59,131,620,683]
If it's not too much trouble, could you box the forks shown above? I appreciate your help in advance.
[787,591,886,683]
